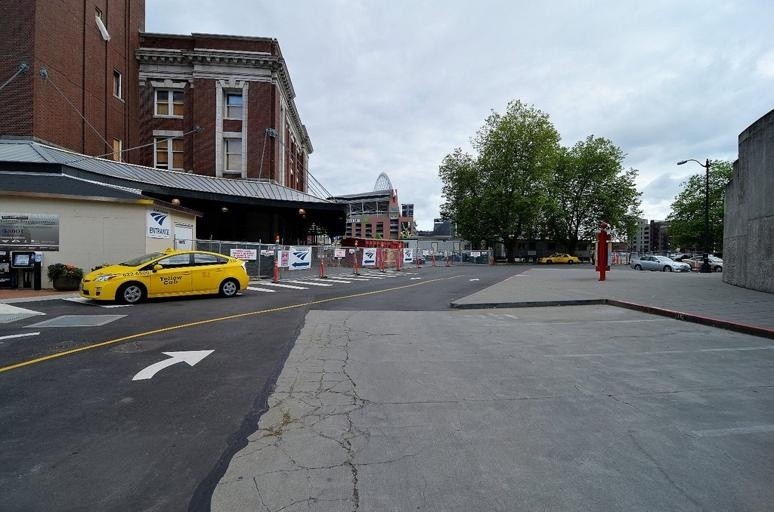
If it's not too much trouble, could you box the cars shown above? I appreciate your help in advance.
[410,252,425,264]
[79,247,249,303]
[707,254,723,263]
[670,253,690,262]
[537,253,580,264]
[682,254,722,272]
[629,255,690,273]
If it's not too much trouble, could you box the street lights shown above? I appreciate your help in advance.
[677,158,710,272]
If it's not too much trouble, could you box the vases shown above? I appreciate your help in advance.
[52,273,81,291]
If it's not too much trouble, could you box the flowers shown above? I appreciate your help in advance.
[46,262,83,282]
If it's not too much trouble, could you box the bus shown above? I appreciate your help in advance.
[337,237,404,268]
